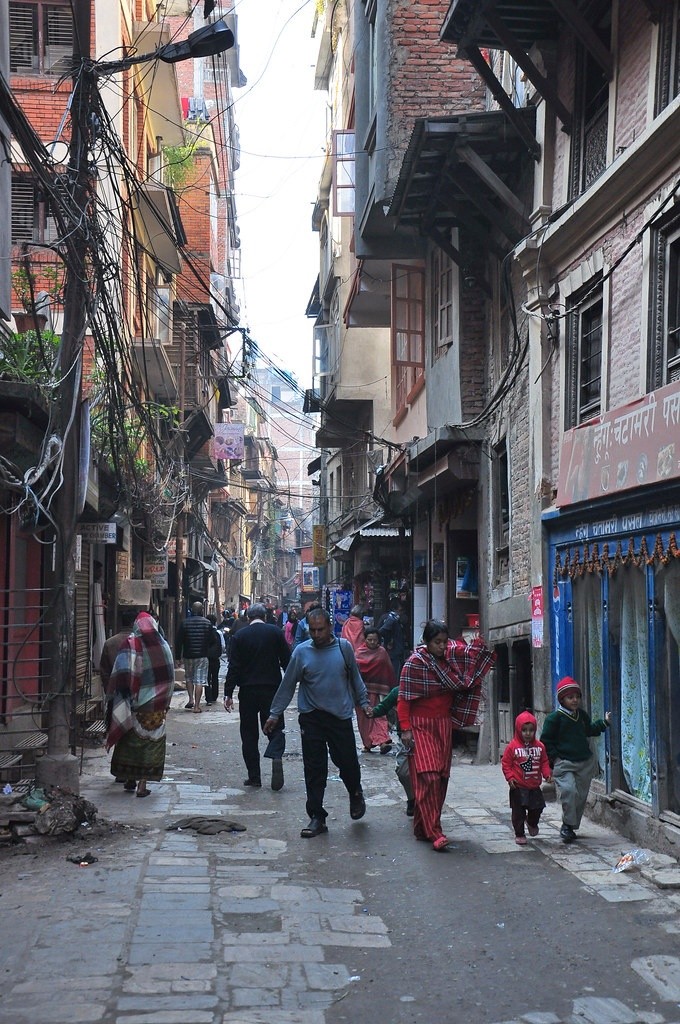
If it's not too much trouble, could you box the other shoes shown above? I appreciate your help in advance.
[380,744,392,755]
[516,835,527,844]
[271,758,284,791]
[524,813,539,837]
[560,825,576,843]
[361,748,370,753]
[244,777,261,788]
[407,799,416,816]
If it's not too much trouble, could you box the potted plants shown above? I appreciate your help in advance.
[8,266,67,336]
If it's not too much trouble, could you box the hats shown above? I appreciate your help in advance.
[556,676,582,702]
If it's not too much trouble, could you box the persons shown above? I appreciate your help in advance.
[502,711,551,845]
[539,677,611,844]
[174,597,405,713]
[262,609,373,837]
[398,619,495,849]
[223,603,293,791]
[368,686,416,816]
[100,609,175,796]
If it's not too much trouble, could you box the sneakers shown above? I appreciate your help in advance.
[300,819,328,838]
[348,784,366,820]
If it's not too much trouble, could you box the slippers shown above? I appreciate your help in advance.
[185,703,193,708]
[137,790,151,797]
[433,837,449,851]
[124,784,136,789]
[193,708,202,713]
